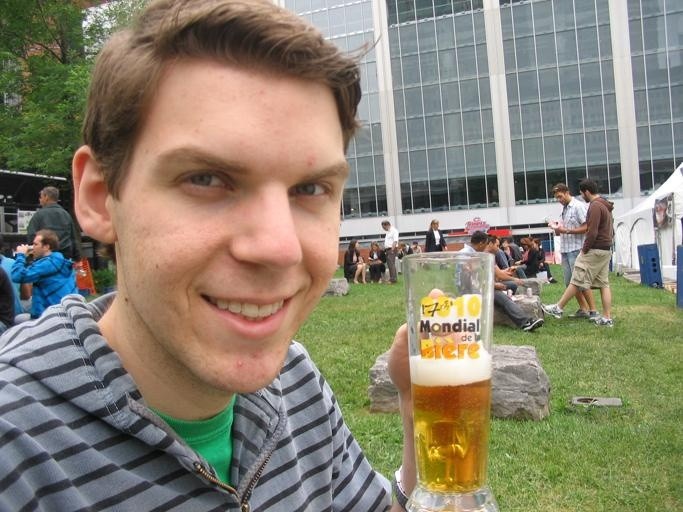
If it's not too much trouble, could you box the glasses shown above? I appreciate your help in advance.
[552,187,561,191]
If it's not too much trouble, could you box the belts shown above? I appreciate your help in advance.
[384,248,390,252]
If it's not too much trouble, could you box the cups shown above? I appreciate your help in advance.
[402,252,499,512]
[358,256,362,264]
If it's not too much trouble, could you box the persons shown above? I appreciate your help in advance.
[11,229,79,325]
[540,180,615,328]
[25,185,81,262]
[0,0,476,512]
[341,219,559,332]
[0,253,29,337]
[546,184,601,321]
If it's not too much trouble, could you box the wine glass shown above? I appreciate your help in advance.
[12,246,33,257]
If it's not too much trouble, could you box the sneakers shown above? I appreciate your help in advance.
[548,278,557,282]
[540,303,563,320]
[568,309,613,327]
[523,318,545,332]
